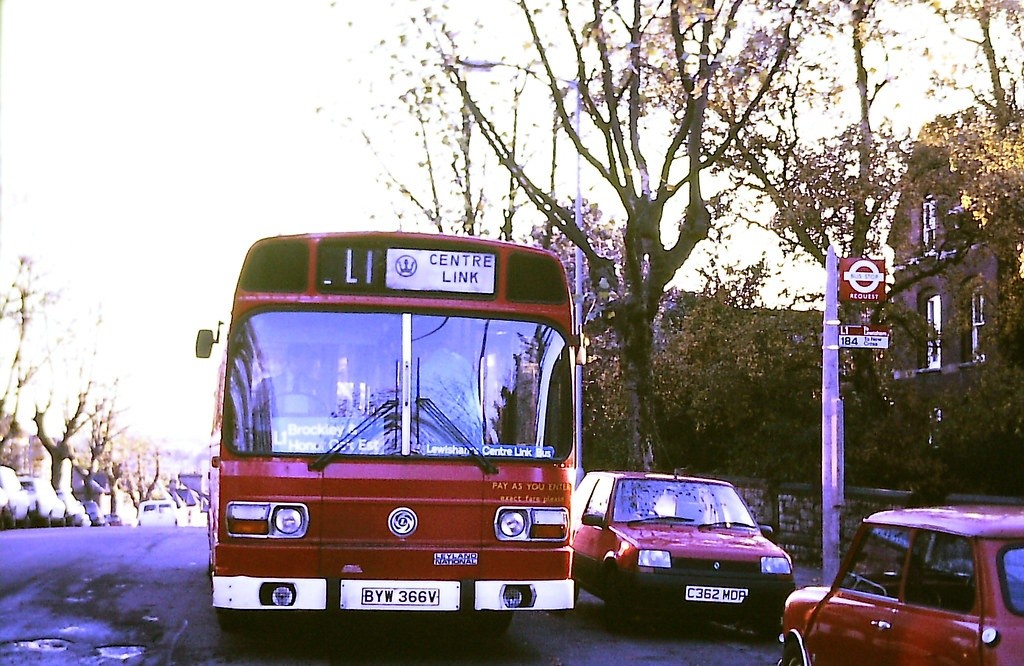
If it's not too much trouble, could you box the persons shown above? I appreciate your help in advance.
[252,341,331,446]
[411,335,486,446]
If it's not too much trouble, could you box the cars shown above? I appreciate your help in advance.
[563,467,799,637]
[778,505,1024,666]
[0,462,210,531]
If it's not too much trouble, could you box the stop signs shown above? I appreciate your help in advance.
[836,258,888,303]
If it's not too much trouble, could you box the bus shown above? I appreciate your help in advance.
[195,227,588,629]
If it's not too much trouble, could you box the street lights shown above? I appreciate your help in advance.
[454,53,588,495]
